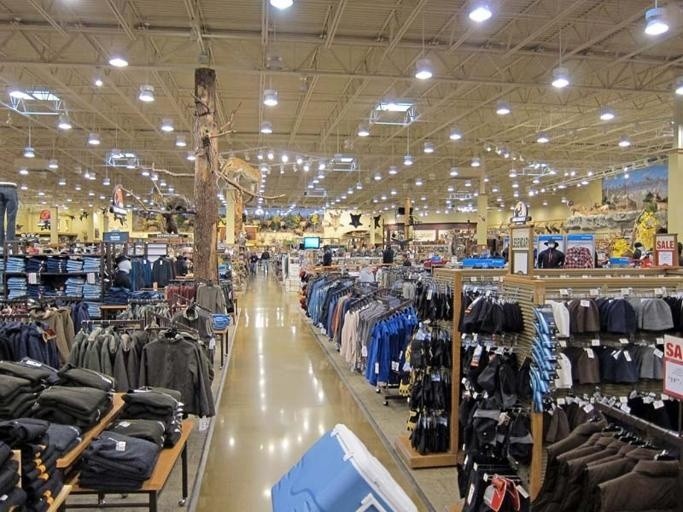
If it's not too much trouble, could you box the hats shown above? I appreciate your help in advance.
[550,296,683,390]
[113,254,131,268]
[544,239,558,247]
[460,292,532,463]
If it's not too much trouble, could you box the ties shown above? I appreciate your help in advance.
[549,249,552,263]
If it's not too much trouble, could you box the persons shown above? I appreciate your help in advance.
[260,250,270,274]
[536,240,564,268]
[402,254,411,266]
[632,242,644,260]
[323,244,331,266]
[0,181,17,246]
[112,254,132,291]
[174,246,195,279]
[382,245,393,264]
[248,251,257,275]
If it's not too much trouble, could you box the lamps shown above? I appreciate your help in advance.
[5,1,227,213]
[252,0,683,217]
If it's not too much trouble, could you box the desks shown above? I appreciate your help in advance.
[46,391,198,510]
